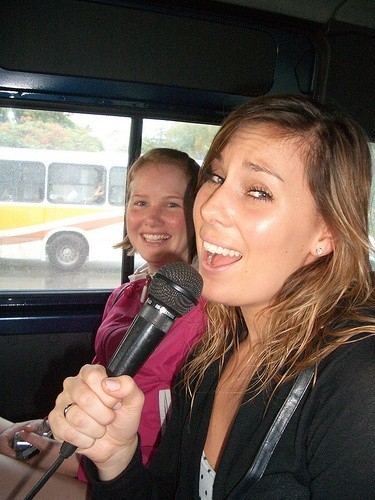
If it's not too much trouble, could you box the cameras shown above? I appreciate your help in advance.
[13,432,48,451]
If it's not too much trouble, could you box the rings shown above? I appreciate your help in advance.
[62,403,77,417]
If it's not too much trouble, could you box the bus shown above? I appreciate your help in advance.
[0,146,205,265]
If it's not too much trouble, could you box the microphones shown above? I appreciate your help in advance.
[59,261,203,458]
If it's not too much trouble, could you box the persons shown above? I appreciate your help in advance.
[0,148,203,500]
[47,91,375,499]
